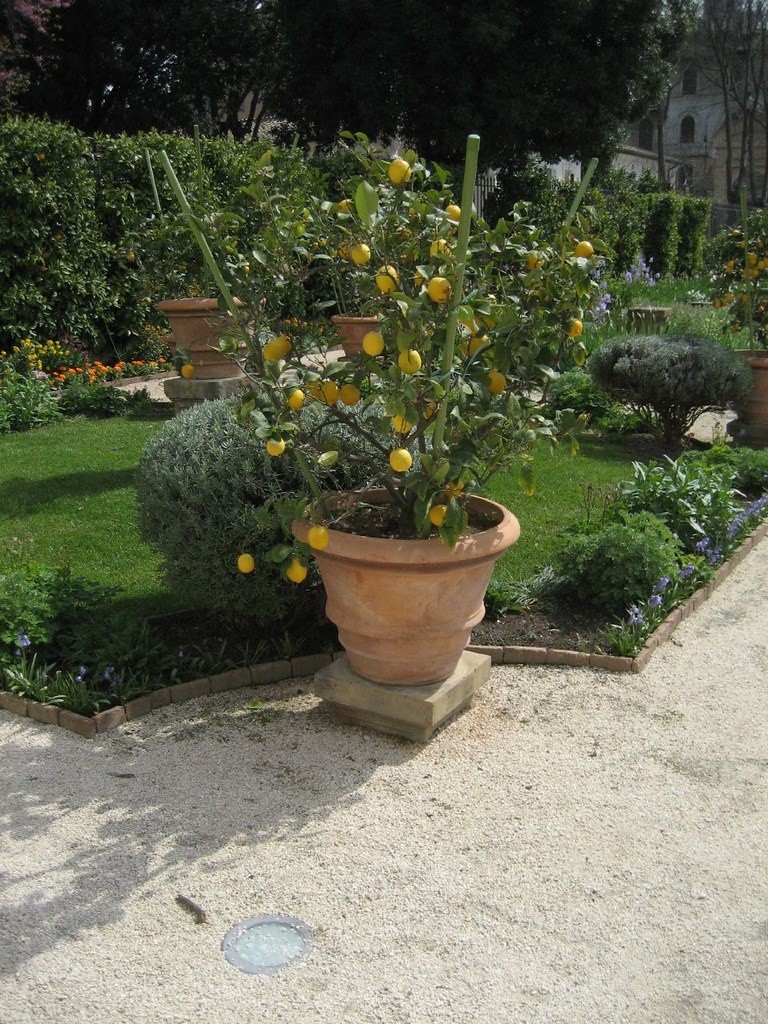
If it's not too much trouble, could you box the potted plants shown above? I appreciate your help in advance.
[125,199,265,379]
[317,202,388,361]
[701,204,768,424]
[159,128,615,686]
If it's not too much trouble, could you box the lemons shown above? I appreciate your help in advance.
[179,160,595,584]
[713,229,768,345]
